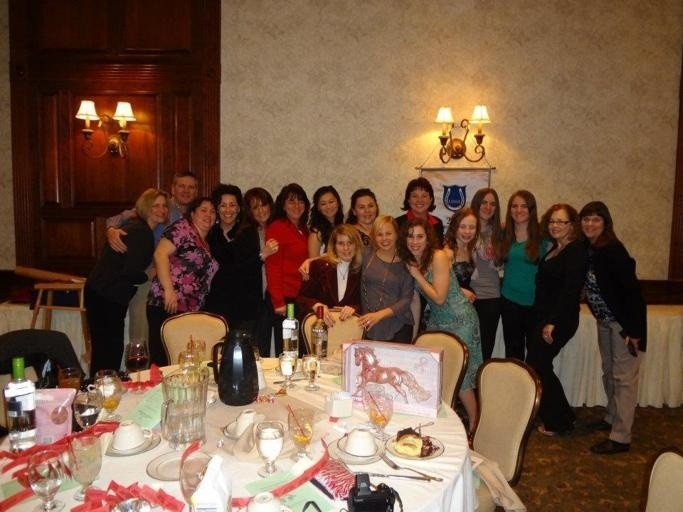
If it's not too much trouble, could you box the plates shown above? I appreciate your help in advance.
[221,416,267,439]
[329,435,442,466]
[146,447,211,481]
[103,428,162,458]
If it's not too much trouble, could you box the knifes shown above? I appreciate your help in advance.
[358,471,431,481]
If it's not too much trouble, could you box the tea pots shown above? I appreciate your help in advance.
[212,330,259,403]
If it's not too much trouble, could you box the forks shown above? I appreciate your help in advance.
[378,453,444,485]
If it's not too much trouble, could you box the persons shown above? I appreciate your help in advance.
[393,177,647,456]
[84,174,414,379]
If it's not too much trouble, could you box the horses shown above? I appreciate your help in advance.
[352,346,432,404]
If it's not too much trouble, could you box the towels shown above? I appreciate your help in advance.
[469,448,527,512]
[191,454,234,512]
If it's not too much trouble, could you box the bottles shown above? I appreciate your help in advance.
[283,302,328,363]
[161,349,210,446]
[2,358,36,451]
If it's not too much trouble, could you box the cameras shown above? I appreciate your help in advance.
[347,473,395,512]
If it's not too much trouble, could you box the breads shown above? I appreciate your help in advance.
[393,429,423,457]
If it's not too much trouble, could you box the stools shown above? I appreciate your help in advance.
[30,282,93,380]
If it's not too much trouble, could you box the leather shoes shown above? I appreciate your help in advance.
[587,418,612,430]
[590,439,631,455]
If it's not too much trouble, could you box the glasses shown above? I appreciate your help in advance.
[547,220,570,225]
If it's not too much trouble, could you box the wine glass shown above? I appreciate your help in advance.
[285,406,318,461]
[124,339,150,396]
[363,385,392,439]
[252,421,283,480]
[70,388,102,433]
[93,369,126,423]
[277,350,297,393]
[300,355,320,393]
[29,452,66,512]
[66,437,101,502]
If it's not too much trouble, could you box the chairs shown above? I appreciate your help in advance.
[300,309,366,359]
[0,329,85,389]
[470,358,542,488]
[645,451,683,512]
[413,330,469,411]
[160,310,230,367]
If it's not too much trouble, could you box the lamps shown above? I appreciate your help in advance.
[435,105,492,164]
[75,100,136,158]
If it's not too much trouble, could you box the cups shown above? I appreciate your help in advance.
[345,428,377,456]
[110,421,154,449]
[236,408,256,437]
[327,394,353,417]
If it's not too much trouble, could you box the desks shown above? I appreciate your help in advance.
[488,302,683,410]
[0,296,130,380]
